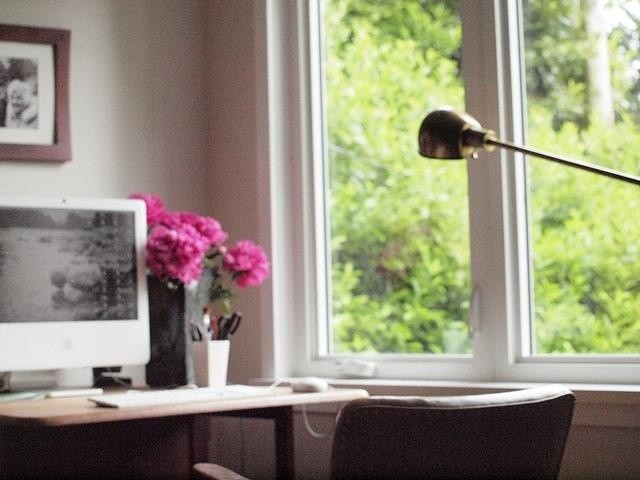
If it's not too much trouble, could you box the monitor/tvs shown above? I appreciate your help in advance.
[0,192,151,398]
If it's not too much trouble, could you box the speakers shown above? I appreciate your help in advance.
[146,275,195,387]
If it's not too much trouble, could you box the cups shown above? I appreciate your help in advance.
[190,340,231,388]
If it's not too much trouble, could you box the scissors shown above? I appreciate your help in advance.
[219,312,241,341]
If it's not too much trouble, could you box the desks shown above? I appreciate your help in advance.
[0,386,371,478]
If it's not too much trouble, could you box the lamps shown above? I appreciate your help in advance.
[417,109,639,188]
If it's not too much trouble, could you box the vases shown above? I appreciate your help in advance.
[147,276,186,389]
[210,290,234,316]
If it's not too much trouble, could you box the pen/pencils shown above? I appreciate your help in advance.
[189,306,221,340]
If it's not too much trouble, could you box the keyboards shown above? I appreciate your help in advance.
[88,384,276,408]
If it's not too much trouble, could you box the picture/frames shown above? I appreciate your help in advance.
[0,23,74,164]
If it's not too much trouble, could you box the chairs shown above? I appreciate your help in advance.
[194,384,578,476]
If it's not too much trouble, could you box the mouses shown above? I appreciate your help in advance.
[292,376,327,393]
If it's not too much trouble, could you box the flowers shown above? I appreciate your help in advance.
[130,192,228,291]
[210,240,269,293]
